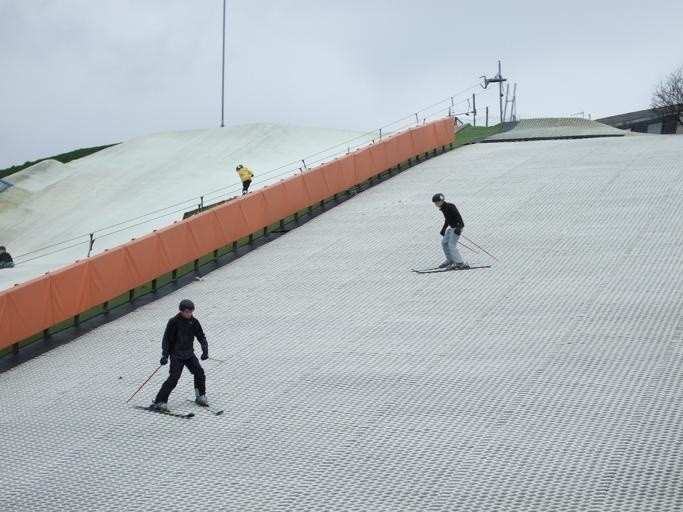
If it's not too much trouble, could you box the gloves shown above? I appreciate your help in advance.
[440,230,445,236]
[454,228,460,234]
[201,354,208,360]
[160,357,168,365]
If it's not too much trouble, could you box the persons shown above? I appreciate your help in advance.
[432,194,464,268]
[236,165,254,195]
[154,300,209,409]
[0,246,13,267]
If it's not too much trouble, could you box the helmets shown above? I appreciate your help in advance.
[179,300,194,311]
[432,193,444,202]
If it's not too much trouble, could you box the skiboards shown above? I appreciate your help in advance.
[412,264,488,274]
[133,394,224,420]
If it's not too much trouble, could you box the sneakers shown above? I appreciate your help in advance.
[439,260,464,269]
[152,399,167,410]
[196,395,207,404]
